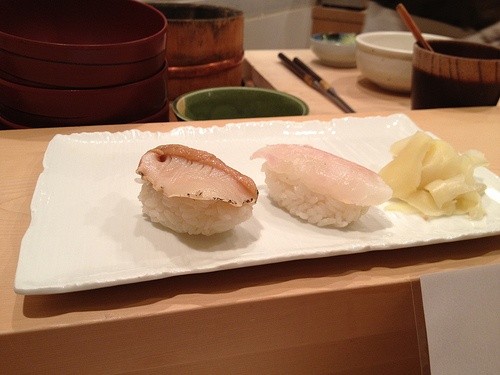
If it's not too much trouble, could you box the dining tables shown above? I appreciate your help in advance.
[3,106,500,375]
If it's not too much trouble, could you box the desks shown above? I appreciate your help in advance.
[244,49,415,115]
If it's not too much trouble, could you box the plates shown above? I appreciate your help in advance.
[13,112,500,297]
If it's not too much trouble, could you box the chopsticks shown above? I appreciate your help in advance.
[280,51,355,115]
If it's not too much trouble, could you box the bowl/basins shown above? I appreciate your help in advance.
[308,31,355,68]
[355,29,457,95]
[172,85,309,122]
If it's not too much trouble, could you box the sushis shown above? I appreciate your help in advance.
[136,142,259,237]
[251,144,392,230]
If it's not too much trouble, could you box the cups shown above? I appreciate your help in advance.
[409,34,499,112]
